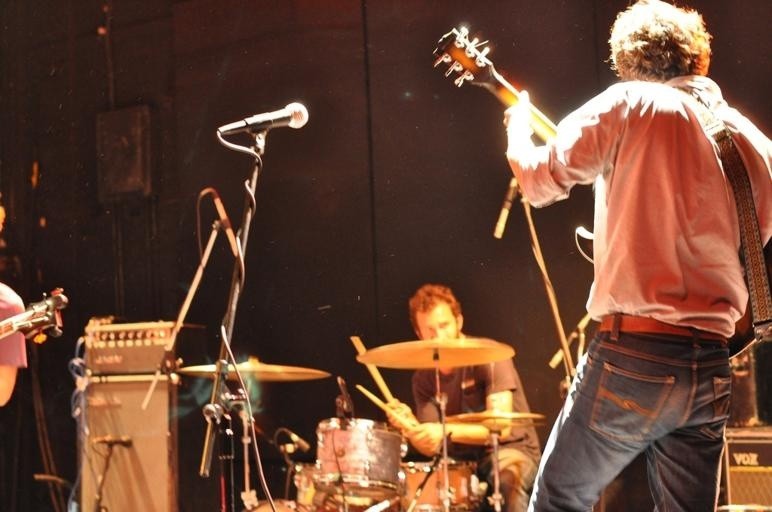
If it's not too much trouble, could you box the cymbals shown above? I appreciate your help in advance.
[446,410,548,425]
[175,363,332,382]
[356,338,516,370]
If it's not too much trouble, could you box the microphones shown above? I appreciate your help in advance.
[546,313,593,370]
[218,101,310,138]
[96,434,133,448]
[492,176,520,240]
[213,193,239,259]
[283,427,311,452]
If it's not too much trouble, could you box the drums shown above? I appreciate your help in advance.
[291,462,374,511]
[316,416,401,496]
[398,461,480,512]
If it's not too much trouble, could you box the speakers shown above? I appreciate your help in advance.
[74,374,221,511]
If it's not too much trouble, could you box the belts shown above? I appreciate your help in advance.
[598,313,728,341]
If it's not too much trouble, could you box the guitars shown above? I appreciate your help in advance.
[432,24,760,360]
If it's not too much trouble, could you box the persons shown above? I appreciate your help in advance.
[499,0,770,511]
[0,279,32,411]
[382,281,543,512]
